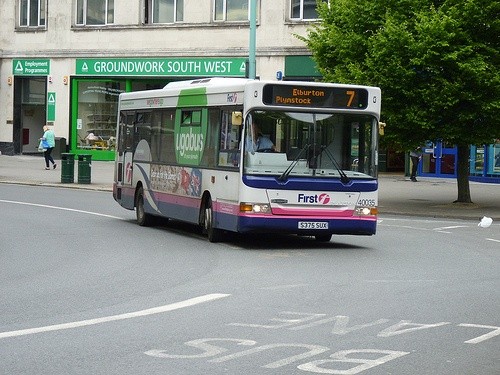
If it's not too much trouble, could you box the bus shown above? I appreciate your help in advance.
[111,75,387,244]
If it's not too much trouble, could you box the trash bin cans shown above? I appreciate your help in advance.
[60,153,76,183]
[77,154,93,184]
[50,137,66,160]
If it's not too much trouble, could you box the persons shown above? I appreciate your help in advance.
[39,125,56,170]
[410,148,421,181]
[238,121,276,152]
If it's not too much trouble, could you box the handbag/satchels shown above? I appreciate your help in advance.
[37,140,50,153]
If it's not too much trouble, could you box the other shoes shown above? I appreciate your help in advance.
[44,167,50,170]
[52,163,57,169]
